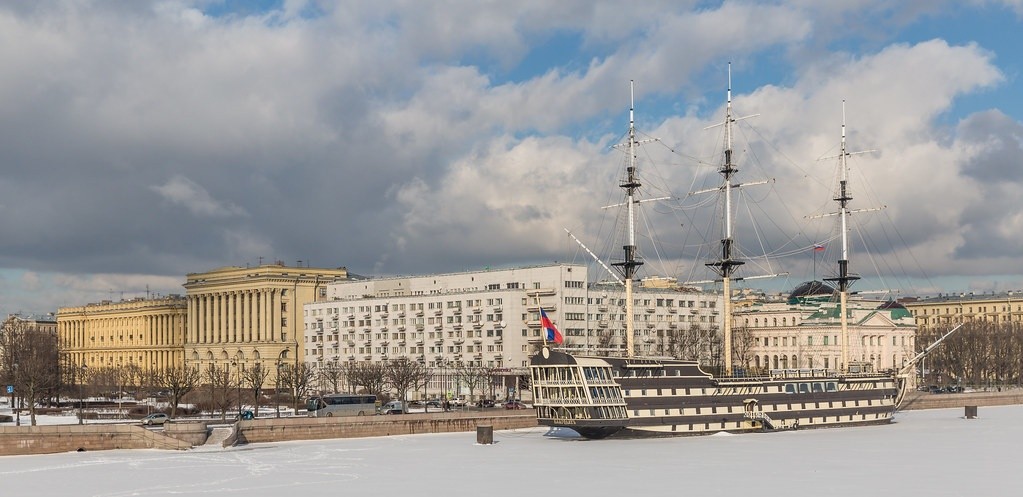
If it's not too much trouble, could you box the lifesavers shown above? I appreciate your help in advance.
[793,423,798,430]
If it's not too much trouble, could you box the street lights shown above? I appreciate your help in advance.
[420,355,427,413]
[232,355,241,421]
[444,357,451,412]
[79,363,88,424]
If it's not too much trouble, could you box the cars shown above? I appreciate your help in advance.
[410,400,441,408]
[918,385,964,394]
[110,392,128,400]
[235,411,255,422]
[142,413,169,426]
[504,402,526,410]
[477,400,495,408]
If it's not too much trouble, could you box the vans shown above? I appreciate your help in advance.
[379,401,408,415]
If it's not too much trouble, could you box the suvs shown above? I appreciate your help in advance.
[448,397,466,406]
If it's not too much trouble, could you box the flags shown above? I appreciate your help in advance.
[814,243,825,252]
[538,306,563,345]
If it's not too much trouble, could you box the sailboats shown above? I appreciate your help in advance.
[526,62,970,438]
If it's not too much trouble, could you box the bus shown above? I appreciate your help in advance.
[304,395,376,418]
